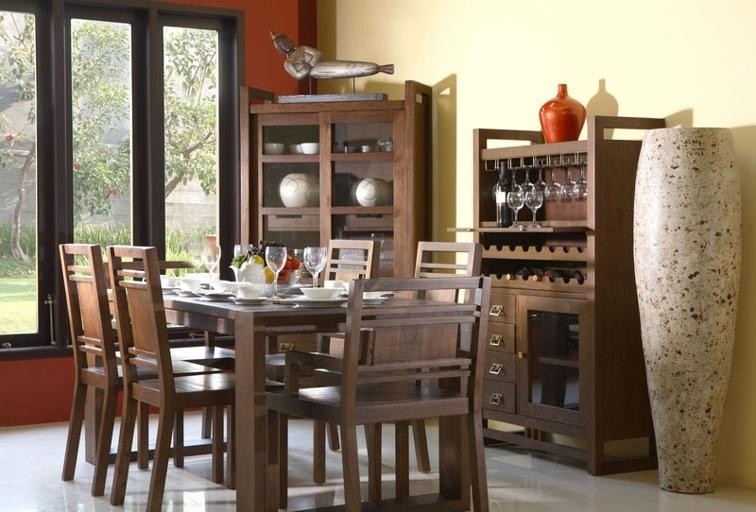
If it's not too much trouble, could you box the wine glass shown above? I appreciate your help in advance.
[303,247,327,287]
[264,246,288,299]
[525,190,544,228]
[506,192,525,228]
[512,166,587,201]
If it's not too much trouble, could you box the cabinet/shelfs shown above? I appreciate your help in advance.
[237,77,432,357]
[472,114,666,475]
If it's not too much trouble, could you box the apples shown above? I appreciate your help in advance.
[264,267,274,284]
[249,255,265,267]
[278,255,299,284]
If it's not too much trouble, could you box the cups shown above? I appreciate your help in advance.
[234,244,253,261]
[362,292,381,298]
[236,287,261,299]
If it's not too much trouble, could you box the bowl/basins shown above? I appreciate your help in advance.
[289,143,319,155]
[263,143,286,155]
[300,287,346,299]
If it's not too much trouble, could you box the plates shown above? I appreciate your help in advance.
[228,297,268,304]
[172,289,202,297]
[294,297,349,308]
[362,297,388,305]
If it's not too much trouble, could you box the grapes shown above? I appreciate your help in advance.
[247,240,285,260]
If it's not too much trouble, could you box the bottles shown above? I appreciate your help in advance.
[495,161,513,228]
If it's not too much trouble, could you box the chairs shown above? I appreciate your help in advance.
[59,238,492,512]
